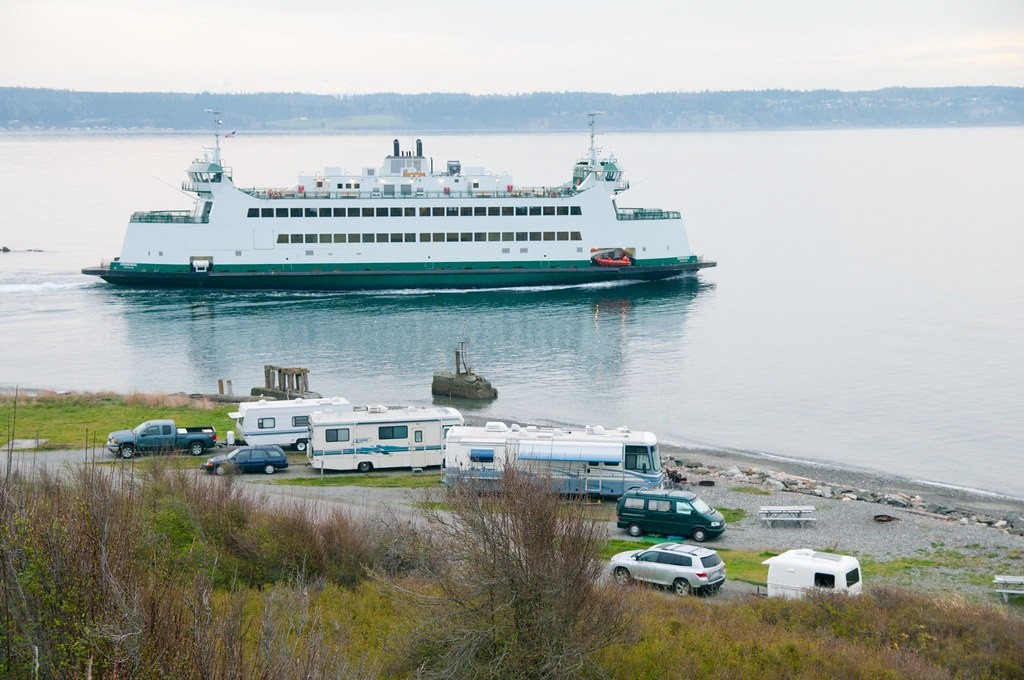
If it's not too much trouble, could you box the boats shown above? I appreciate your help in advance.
[79,108,716,291]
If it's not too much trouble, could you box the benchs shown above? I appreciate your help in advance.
[759,511,817,528]
[996,589,1024,602]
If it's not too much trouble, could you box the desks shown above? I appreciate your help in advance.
[993,575,1024,603]
[760,505,816,527]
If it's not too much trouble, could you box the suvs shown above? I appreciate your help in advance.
[610,543,727,597]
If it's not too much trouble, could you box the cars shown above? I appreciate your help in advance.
[203,445,289,476]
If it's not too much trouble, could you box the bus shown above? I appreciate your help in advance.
[442,421,664,497]
[306,406,465,472]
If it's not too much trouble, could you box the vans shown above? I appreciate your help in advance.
[228,398,352,452]
[616,487,726,543]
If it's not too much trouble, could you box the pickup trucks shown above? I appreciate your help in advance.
[106,420,217,460]
